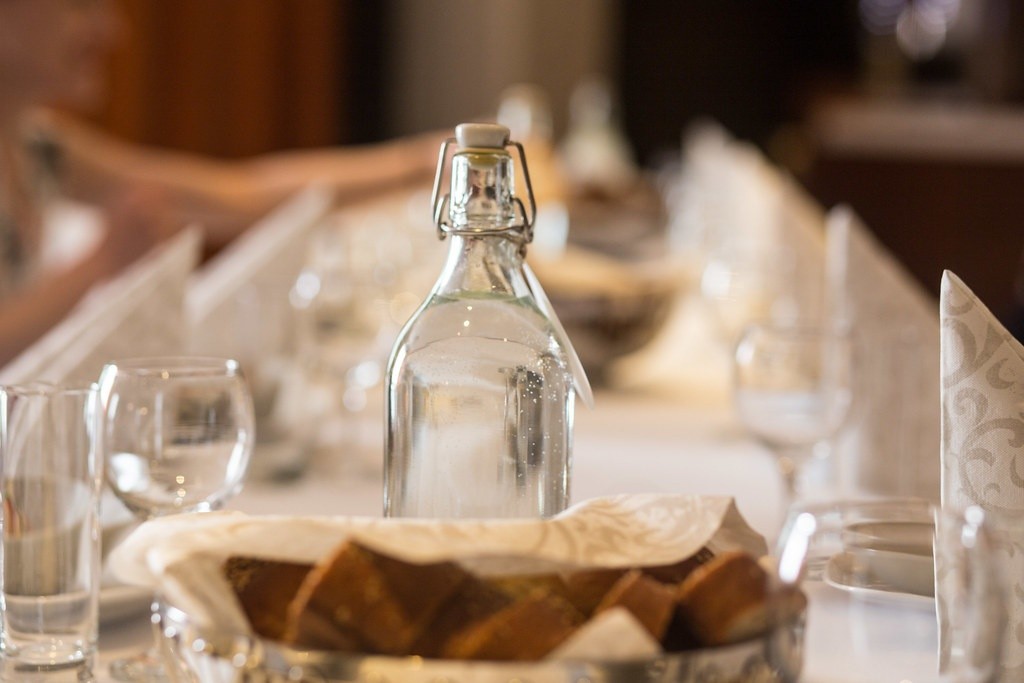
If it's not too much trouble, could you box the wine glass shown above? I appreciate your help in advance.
[735,327,856,544]
[99,360,256,683]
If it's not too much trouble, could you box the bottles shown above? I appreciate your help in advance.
[380,146,595,519]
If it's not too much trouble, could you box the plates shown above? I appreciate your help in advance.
[824,553,935,611]
[845,520,937,556]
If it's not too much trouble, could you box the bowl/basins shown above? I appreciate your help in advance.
[148,581,811,683]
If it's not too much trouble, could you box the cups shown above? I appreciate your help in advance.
[0,379,104,683]
